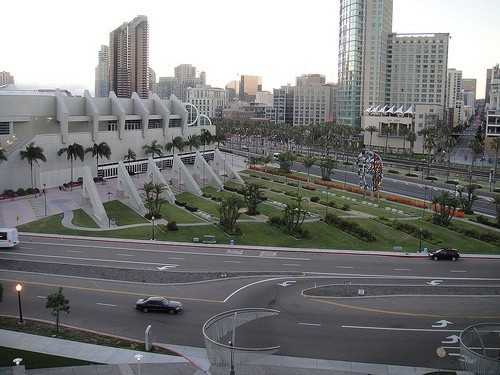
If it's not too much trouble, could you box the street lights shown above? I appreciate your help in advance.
[152,216,155,240]
[16,283,24,323]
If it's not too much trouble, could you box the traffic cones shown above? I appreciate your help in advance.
[29,237,32,242]
[59,236,64,244]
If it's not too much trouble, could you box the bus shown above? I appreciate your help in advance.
[0,227,20,247]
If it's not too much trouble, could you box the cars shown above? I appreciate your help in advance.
[242,145,249,149]
[134,296,184,315]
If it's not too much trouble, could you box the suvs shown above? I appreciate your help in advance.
[428,247,460,262]
